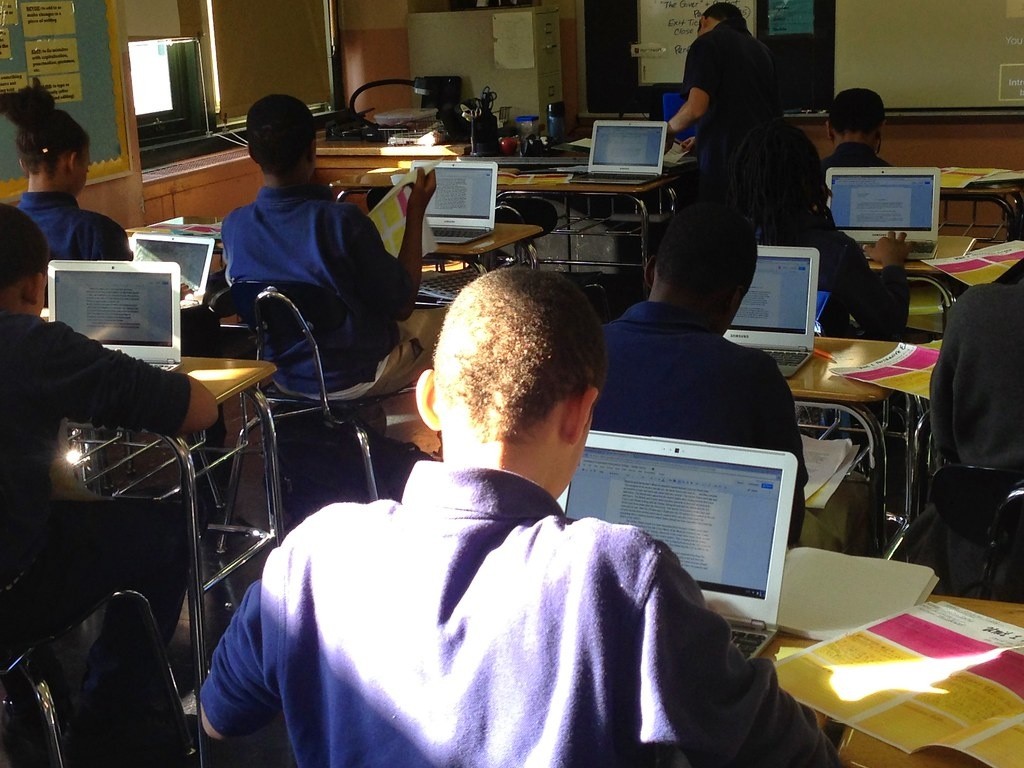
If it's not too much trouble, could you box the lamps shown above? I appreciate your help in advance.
[348,75,439,133]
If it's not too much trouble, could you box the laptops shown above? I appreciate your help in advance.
[410,160,498,244]
[723,245,819,378]
[824,167,941,260]
[129,233,215,309]
[568,120,668,185]
[556,429,798,664]
[47,260,182,372]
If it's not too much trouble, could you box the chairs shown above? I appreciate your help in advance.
[0,179,1024,768]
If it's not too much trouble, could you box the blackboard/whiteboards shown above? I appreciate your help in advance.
[0,0,133,204]
[636,0,757,86]
[576,0,837,116]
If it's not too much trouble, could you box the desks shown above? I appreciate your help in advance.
[164,137,1024,767]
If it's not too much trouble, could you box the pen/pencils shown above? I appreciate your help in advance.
[814,349,835,360]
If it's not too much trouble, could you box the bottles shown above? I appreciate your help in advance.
[473,99,497,156]
[546,101,565,145]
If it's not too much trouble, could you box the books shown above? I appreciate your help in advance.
[367,156,439,257]
[775,544,940,642]
[773,601,1024,768]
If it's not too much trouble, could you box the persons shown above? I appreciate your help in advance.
[199,261,846,768]
[817,89,894,174]
[221,94,436,435]
[903,275,1024,604]
[0,82,133,306]
[590,201,808,548]
[0,204,219,768]
[725,117,914,341]
[663,2,783,202]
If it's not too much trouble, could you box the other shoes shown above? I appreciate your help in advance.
[1,696,56,764]
[60,702,200,767]
